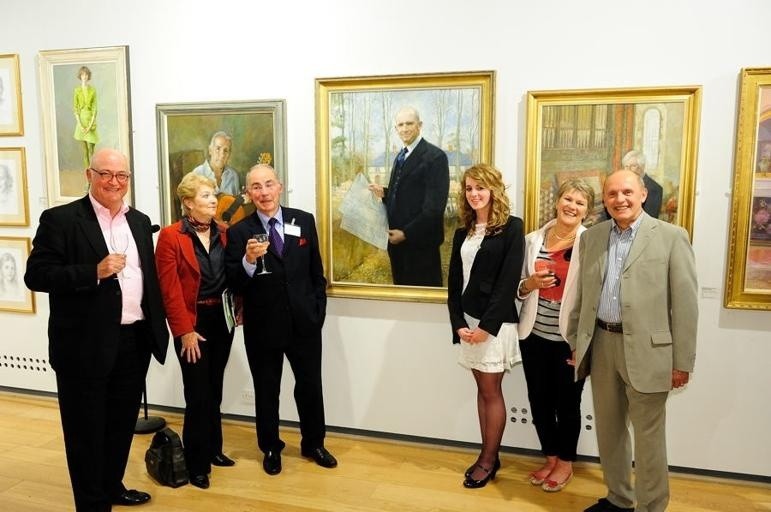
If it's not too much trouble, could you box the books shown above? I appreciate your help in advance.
[221,287,236,333]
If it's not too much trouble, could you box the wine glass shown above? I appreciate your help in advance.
[253,232,273,277]
[543,260,561,307]
[110,228,130,281]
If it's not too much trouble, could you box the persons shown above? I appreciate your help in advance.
[515,176,594,493]
[73,66,99,168]
[366,108,450,287]
[0,252,20,297]
[0,164,16,211]
[573,164,699,511]
[221,162,338,475]
[445,165,525,487]
[155,173,237,488]
[188,129,252,228]
[23,151,170,512]
[603,151,664,220]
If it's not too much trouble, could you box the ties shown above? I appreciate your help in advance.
[390,148,408,210]
[267,218,285,258]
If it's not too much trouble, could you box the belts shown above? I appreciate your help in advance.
[597,318,623,334]
[198,297,222,306]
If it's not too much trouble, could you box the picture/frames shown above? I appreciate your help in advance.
[156,100,288,232]
[36,45,137,212]
[724,65,771,311]
[314,69,497,303]
[1,235,36,314]
[0,53,26,138]
[0,147,31,228]
[524,84,703,246]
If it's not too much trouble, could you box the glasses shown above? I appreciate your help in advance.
[89,167,131,183]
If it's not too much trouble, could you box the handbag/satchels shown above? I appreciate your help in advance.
[146,427,190,488]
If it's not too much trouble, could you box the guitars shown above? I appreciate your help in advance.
[213,152,272,229]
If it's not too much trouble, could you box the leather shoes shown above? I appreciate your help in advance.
[302,447,337,467]
[263,449,282,475]
[584,499,634,512]
[210,454,235,466]
[192,471,209,488]
[530,466,574,492]
[112,490,150,505]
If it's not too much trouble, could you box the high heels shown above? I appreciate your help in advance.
[464,453,501,488]
